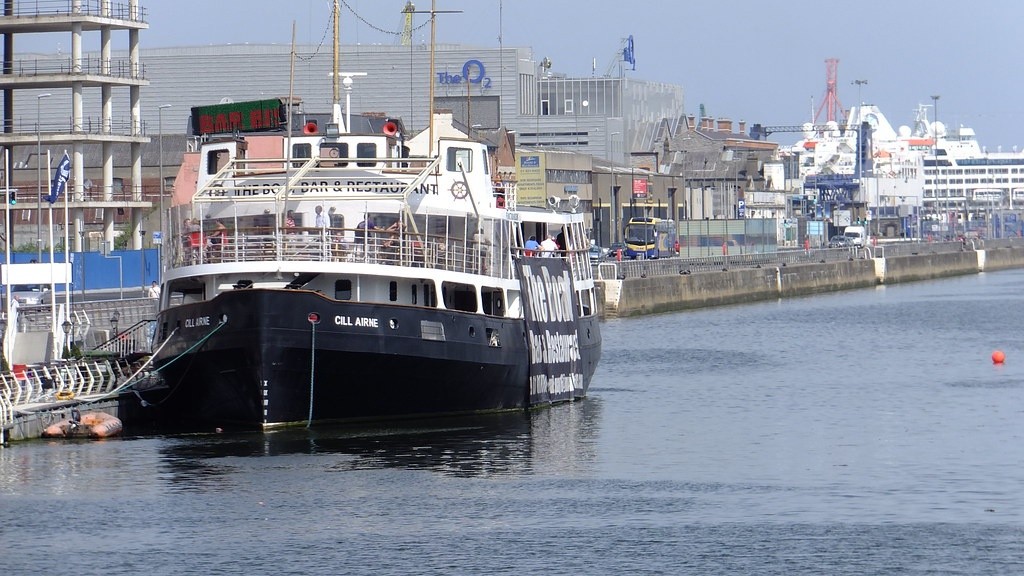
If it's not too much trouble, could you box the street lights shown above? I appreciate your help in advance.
[850,78,869,225]
[158,104,173,294]
[931,93,940,239]
[326,71,367,137]
[36,93,54,261]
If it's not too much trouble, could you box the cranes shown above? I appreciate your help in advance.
[756,124,873,176]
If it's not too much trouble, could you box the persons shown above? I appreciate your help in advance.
[11,205,572,329]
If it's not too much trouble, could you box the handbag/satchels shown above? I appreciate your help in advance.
[211,234,221,244]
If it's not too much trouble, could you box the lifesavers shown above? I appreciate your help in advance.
[56,391,75,401]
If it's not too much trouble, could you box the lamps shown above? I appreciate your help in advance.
[325,122,339,138]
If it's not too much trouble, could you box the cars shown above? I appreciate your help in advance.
[608,242,626,257]
[10,284,52,307]
[829,235,853,249]
[585,246,605,265]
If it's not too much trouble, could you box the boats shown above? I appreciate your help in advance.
[42,410,122,439]
[106,138,605,431]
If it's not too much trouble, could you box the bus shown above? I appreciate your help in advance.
[623,217,679,259]
[843,226,866,248]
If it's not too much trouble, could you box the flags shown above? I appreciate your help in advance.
[47,151,71,202]
